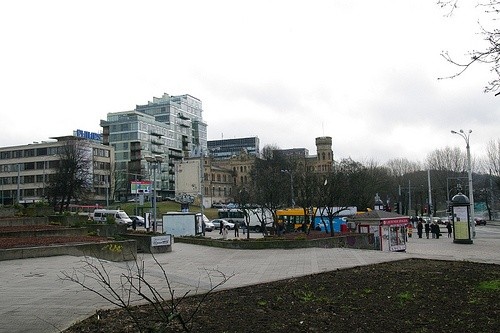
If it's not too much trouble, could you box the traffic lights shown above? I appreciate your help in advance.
[387,201,391,212]
[428,204,434,214]
[394,202,398,214]
[424,207,427,213]
[383,204,387,211]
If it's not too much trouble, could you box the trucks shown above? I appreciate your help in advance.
[167,211,214,232]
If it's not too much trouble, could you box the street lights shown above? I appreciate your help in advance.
[450,128,476,238]
[401,172,411,217]
[281,169,295,210]
[41,153,57,207]
[144,155,163,234]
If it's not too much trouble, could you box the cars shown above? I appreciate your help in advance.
[468,216,487,227]
[210,218,235,230]
[410,215,453,225]
[129,216,145,226]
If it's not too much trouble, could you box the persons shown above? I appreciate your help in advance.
[132,218,136,229]
[411,216,440,239]
[152,222,157,231]
[241,221,246,234]
[316,224,322,231]
[219,221,228,239]
[446,223,452,238]
[234,222,240,237]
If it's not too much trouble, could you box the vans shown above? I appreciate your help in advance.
[93,209,133,227]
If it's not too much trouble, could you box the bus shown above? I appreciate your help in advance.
[54,204,103,221]
[216,205,372,234]
